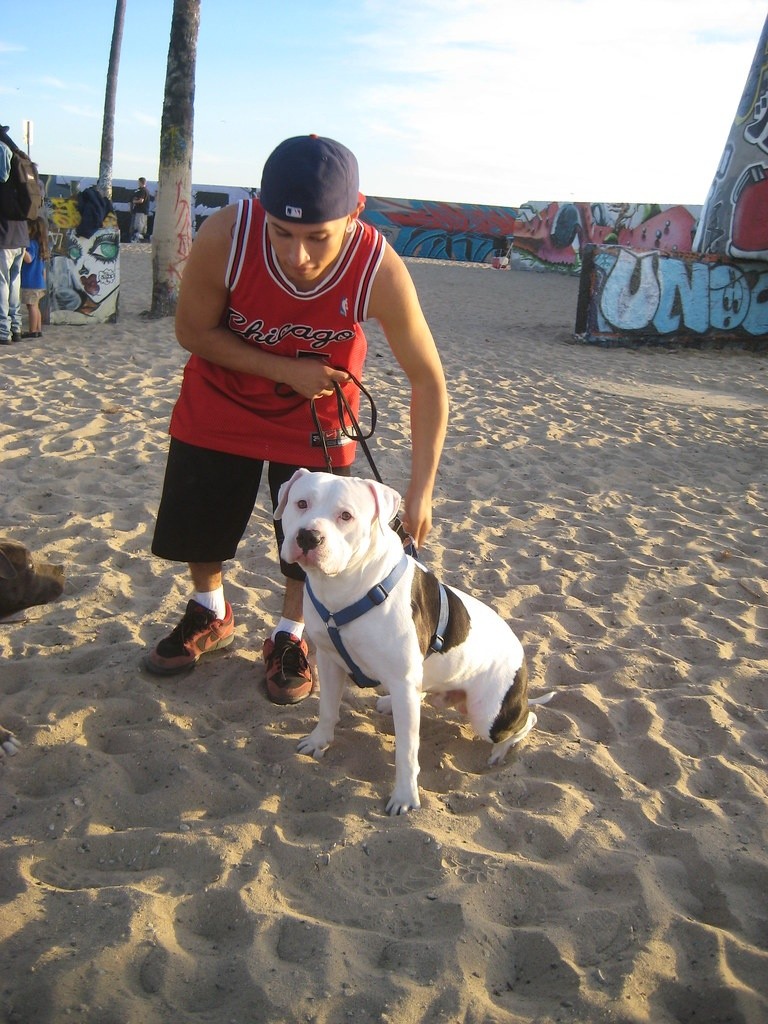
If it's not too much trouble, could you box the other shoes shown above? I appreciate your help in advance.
[22,332,42,338]
[13,333,22,342]
[0,340,12,345]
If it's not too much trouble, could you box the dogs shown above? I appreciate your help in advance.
[272,467,538,818]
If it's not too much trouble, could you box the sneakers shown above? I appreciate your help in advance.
[144,600,235,675]
[264,631,313,704]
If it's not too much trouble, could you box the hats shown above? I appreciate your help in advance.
[262,135,366,221]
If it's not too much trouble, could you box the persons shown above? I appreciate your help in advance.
[130,176,148,243]
[144,133,449,704]
[0,122,46,346]
